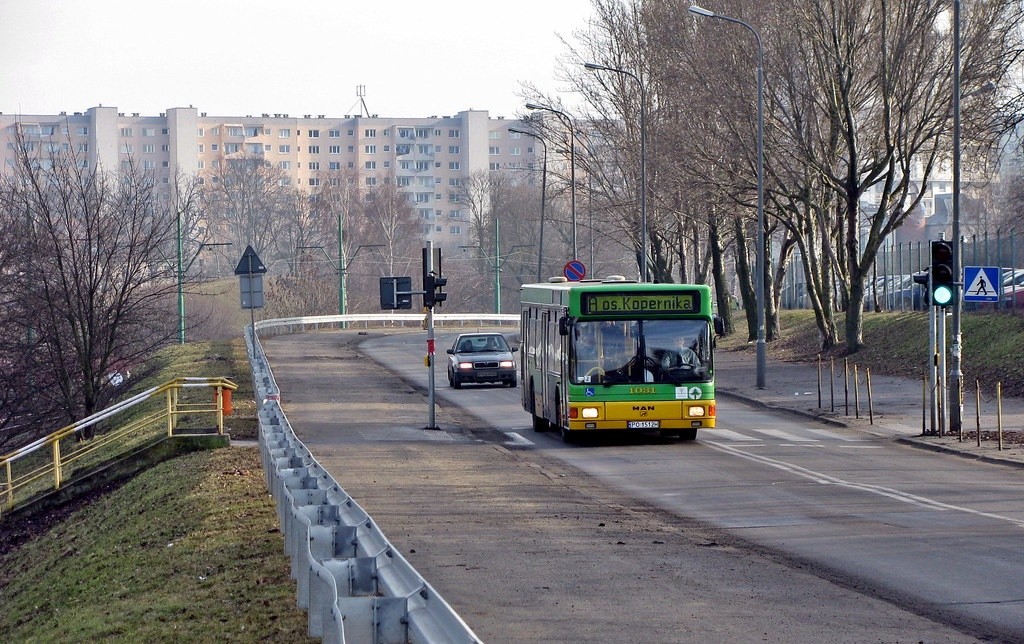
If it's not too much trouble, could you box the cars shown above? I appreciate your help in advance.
[780,278,843,310]
[447,332,519,389]
[863,270,929,312]
[960,266,1024,308]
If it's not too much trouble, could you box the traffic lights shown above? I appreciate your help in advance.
[913,273,932,289]
[426,275,448,306]
[932,241,953,305]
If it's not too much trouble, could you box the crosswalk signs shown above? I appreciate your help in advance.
[963,266,1001,303]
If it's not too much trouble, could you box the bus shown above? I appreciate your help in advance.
[520,275,726,442]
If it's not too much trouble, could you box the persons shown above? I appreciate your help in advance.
[662,334,702,368]
[484,336,500,349]
[460,340,476,350]
[575,321,631,378]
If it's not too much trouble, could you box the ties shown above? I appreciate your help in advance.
[677,351,682,366]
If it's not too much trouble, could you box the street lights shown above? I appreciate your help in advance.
[687,5,765,390]
[584,61,647,282]
[506,126,547,283]
[525,103,577,260]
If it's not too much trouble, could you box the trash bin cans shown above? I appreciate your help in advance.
[213,377,232,416]
